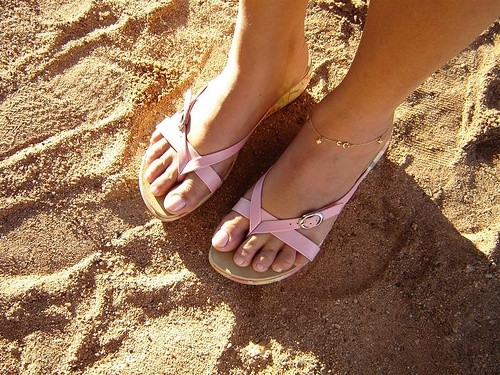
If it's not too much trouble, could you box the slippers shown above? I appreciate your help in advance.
[139,48,312,222]
[209,105,394,285]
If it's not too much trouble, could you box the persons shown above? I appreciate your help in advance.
[139,0,500,285]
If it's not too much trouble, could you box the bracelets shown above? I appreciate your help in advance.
[306,109,392,150]
